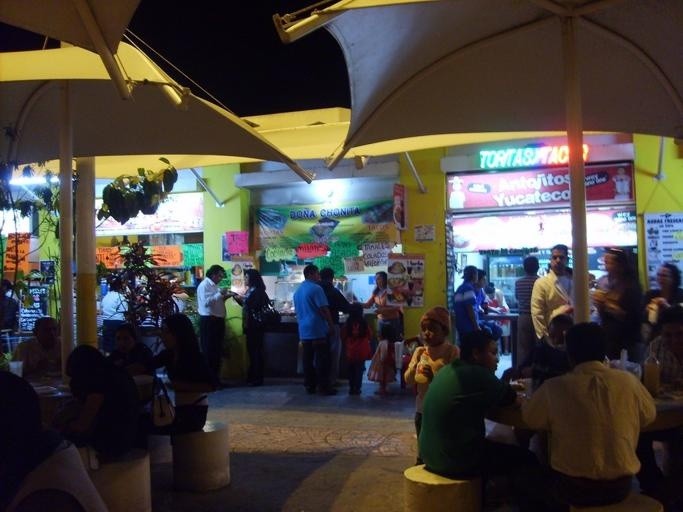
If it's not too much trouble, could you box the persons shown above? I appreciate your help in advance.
[195,265,236,390]
[291,263,402,397]
[231,268,272,384]
[1,279,211,512]
[402,305,461,465]
[453,243,682,370]
[519,320,657,509]
[415,328,539,512]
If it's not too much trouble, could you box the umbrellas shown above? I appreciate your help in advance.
[0,0,145,103]
[0,39,313,381]
[274,0,682,337]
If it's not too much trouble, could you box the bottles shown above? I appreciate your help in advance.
[644,351,660,394]
[490,264,523,289]
[484,298,489,314]
[415,351,429,383]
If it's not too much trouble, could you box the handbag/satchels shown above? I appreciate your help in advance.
[248,301,277,333]
[152,378,176,427]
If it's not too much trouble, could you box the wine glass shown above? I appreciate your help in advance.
[406,266,414,306]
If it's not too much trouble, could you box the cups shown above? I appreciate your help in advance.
[648,303,658,323]
[9,362,22,378]
[516,378,532,399]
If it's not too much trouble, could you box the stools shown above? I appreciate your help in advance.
[569,492,664,512]
[93,448,152,511]
[170,421,231,491]
[404,464,482,512]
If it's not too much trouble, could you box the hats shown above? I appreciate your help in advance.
[421,306,450,335]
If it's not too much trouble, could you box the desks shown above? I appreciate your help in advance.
[481,312,519,368]
